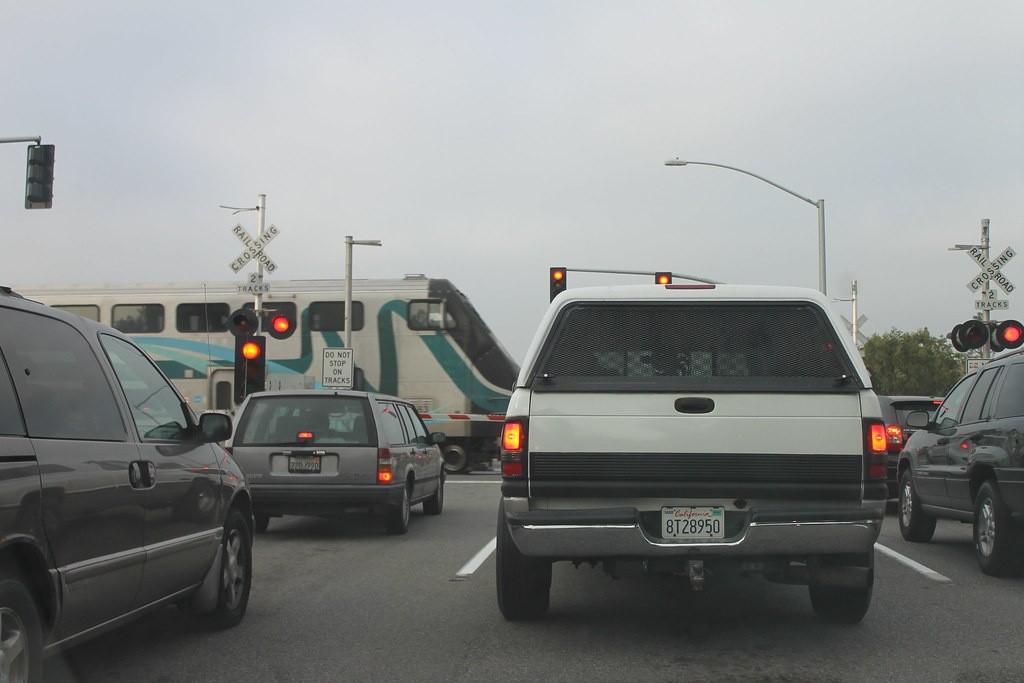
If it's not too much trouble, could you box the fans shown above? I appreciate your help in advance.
[650,347,692,377]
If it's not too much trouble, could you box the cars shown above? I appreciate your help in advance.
[224,388,446,535]
[493,285,891,625]
[1,281,254,682]
[877,395,945,511]
[894,351,1023,572]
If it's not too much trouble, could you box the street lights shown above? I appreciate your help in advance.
[664,157,828,298]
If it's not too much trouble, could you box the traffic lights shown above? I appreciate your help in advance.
[234,335,265,405]
[950,319,988,351]
[655,271,671,285]
[991,319,1022,354]
[548,266,567,304]
[261,310,296,339]
[229,308,256,337]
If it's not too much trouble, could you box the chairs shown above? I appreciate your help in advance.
[305,409,334,437]
[548,367,621,377]
[343,417,367,442]
[267,416,311,442]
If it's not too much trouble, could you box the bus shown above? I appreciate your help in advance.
[1,275,524,498]
